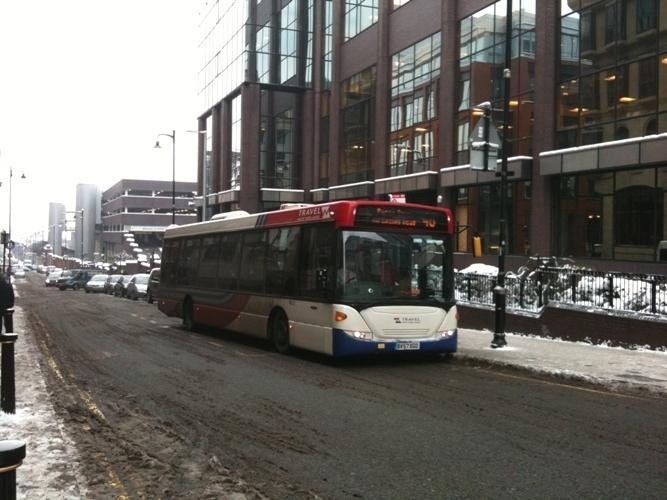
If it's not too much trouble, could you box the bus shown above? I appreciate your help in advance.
[156,193,482,363]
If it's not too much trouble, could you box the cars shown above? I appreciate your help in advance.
[86,274,149,302]
[10,259,62,278]
[45,273,62,286]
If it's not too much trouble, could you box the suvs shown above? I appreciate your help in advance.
[147,268,162,304]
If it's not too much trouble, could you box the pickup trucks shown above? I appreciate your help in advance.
[57,270,92,292]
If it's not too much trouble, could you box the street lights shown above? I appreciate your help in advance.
[65,209,86,270]
[492,0,513,347]
[22,225,56,265]
[0,168,26,273]
[153,129,176,226]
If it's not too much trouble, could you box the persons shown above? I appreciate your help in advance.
[0,271,10,334]
